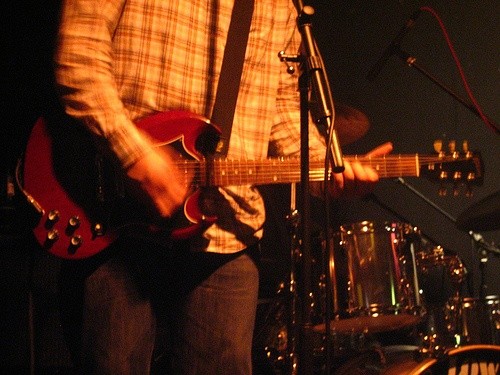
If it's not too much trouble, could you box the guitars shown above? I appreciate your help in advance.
[23,106,487,261]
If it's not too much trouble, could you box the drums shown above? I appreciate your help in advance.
[328,342,500,375]
[433,291,500,346]
[292,218,428,337]
[416,245,478,300]
[251,295,292,370]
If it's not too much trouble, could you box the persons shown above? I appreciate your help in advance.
[53,0,379,374]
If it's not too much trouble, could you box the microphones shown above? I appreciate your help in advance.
[368,8,422,81]
